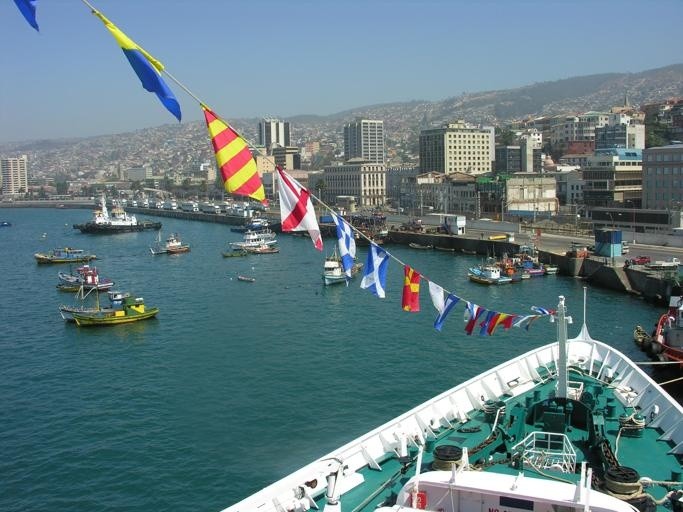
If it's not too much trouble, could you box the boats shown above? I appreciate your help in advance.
[312,206,559,285]
[147,230,191,255]
[1,219,13,230]
[221,212,284,264]
[632,281,681,382]
[235,274,256,284]
[215,281,682,512]
[29,230,163,334]
[73,197,162,233]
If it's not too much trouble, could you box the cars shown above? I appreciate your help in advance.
[630,256,650,265]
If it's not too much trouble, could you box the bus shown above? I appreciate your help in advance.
[620,240,628,255]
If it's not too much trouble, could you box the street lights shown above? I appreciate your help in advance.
[624,199,637,244]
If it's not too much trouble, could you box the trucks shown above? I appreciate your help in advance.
[94,192,256,216]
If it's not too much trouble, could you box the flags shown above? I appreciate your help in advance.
[275,164,558,337]
[12,0,39,32]
[91,10,181,125]
[200,103,270,209]
